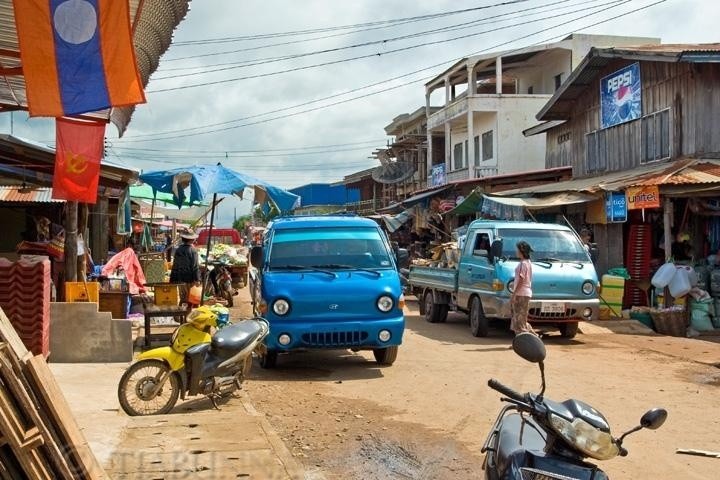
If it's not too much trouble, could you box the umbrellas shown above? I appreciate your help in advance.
[138,161,302,305]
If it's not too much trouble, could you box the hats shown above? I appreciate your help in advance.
[179,226,200,239]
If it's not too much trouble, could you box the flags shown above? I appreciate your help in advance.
[51,117,107,206]
[11,0,147,118]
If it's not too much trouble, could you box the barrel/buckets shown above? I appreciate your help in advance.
[651,263,676,287]
[677,264,698,287]
[667,266,692,299]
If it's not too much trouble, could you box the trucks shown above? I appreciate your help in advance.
[249,215,405,363]
[196,228,250,286]
[410,218,601,338]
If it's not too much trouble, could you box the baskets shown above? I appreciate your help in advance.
[651,308,689,339]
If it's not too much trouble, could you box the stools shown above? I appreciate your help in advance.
[623,224,651,309]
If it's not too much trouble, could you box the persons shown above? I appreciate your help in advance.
[169,228,203,322]
[507,241,540,349]
[579,228,593,252]
[165,233,172,262]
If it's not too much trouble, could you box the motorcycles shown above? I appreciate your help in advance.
[480,332,667,480]
[201,260,237,306]
[117,302,269,416]
